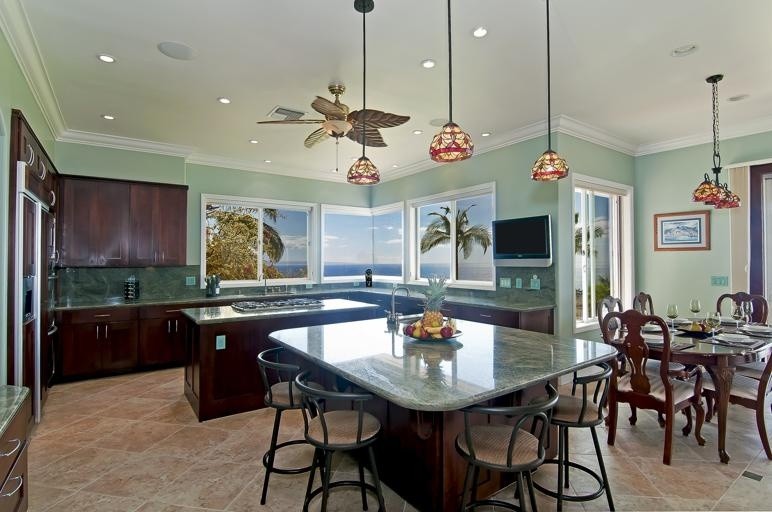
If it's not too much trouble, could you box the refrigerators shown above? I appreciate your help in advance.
[36,201,57,334]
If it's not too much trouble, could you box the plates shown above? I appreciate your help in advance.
[640,317,772,345]
[401,327,463,342]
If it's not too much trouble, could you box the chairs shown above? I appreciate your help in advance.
[455,384,559,512]
[597,292,772,465]
[528,362,615,512]
[295,370,385,512]
[257,347,335,505]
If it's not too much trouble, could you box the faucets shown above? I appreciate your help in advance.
[391,286,410,313]
[259,273,267,295]
[392,325,410,359]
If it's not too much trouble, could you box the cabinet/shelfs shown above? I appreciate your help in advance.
[0,385,30,512]
[138,306,187,372]
[57,178,131,266]
[54,308,138,376]
[129,181,188,268]
[356,295,554,334]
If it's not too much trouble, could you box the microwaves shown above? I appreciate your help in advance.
[21,277,37,323]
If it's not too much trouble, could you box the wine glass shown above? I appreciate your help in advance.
[730,305,744,333]
[690,299,701,323]
[666,302,679,332]
[741,302,754,327]
[705,313,722,345]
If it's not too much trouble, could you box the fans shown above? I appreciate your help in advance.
[256,84,411,172]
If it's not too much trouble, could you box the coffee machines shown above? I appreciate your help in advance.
[206,274,220,298]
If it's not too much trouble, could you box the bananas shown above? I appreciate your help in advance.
[424,327,442,338]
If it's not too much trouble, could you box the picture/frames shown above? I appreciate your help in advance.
[654,210,710,251]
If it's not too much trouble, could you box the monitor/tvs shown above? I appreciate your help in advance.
[492,214,553,268]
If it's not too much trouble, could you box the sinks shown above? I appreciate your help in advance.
[401,316,449,325]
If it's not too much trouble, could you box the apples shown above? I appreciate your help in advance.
[440,326,454,337]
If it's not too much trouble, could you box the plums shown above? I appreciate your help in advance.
[407,326,415,336]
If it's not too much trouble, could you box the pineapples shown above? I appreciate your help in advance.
[423,312,443,327]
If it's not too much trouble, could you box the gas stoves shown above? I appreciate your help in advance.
[231,298,325,311]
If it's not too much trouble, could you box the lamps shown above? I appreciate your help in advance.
[531,0,569,181]
[429,0,474,162]
[691,75,741,208]
[347,0,380,184]
